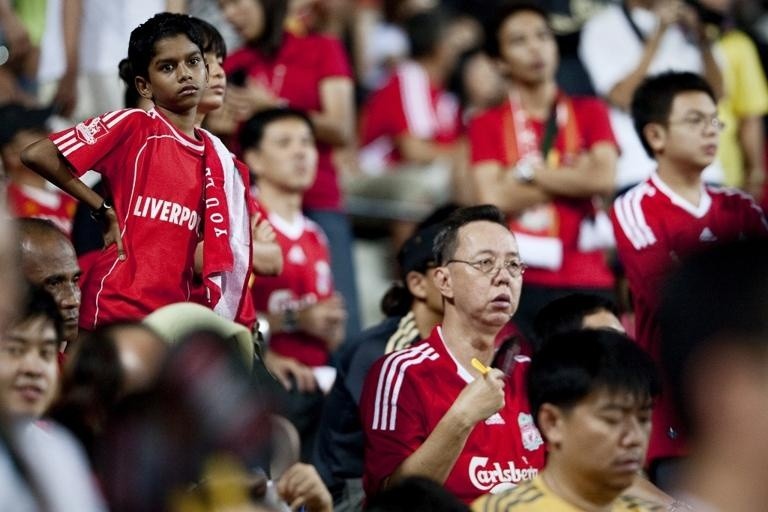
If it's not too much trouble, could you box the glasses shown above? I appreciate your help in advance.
[445,258,527,277]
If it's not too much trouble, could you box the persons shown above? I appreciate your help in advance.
[0,0,361,512]
[354,202,683,512]
[646,221,767,512]
[356,2,767,345]
